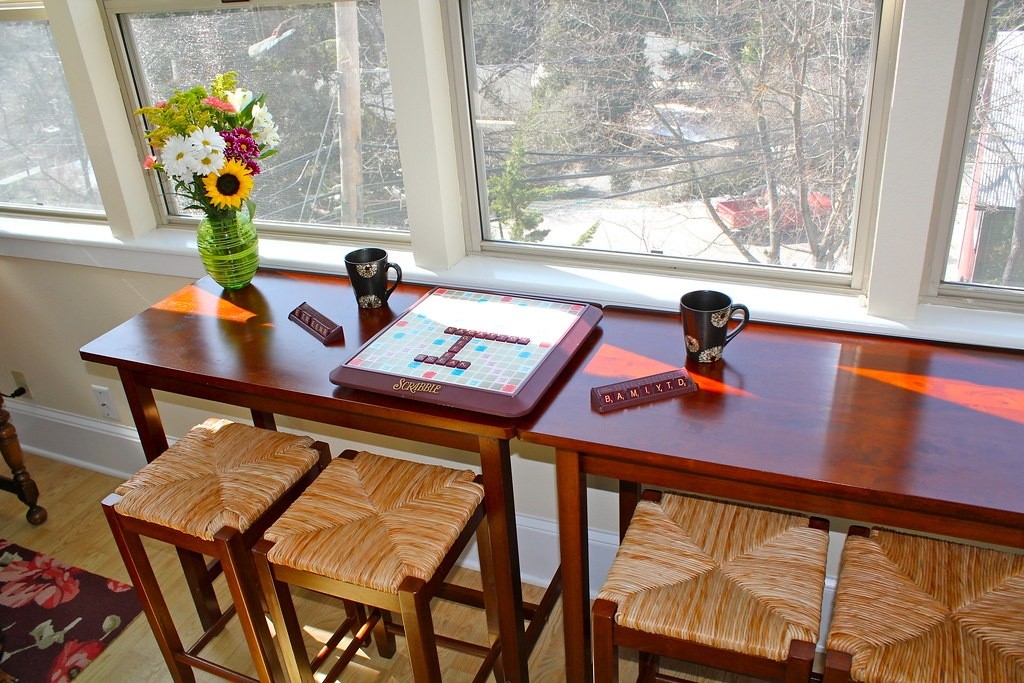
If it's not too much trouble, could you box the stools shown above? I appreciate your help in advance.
[101,417,372,683]
[592,488,831,683]
[0,392,47,525]
[823,525,1024,683]
[251,450,505,683]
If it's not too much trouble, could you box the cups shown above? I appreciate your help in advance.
[680,291,749,363]
[344,248,402,309]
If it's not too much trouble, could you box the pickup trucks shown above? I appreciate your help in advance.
[715,189,832,232]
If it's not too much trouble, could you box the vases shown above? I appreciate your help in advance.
[196,210,259,291]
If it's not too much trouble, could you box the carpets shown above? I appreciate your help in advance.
[0,539,142,683]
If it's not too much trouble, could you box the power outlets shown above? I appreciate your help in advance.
[10,370,32,400]
[90,384,121,422]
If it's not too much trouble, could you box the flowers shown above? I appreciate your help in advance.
[131,70,280,223]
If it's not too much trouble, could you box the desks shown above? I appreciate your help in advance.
[516,305,1024,683]
[79,267,602,683]
